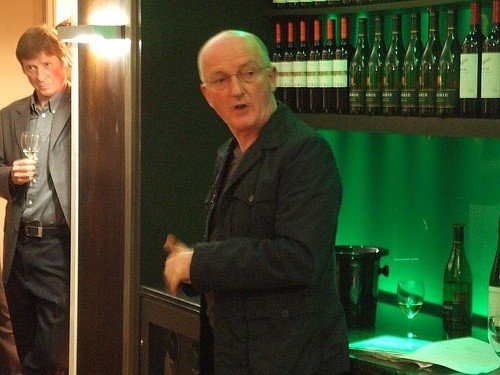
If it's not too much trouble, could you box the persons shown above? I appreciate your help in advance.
[0,19,73,375]
[163,31,352,375]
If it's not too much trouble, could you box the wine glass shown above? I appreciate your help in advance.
[20,132,40,180]
[396,281,423,338]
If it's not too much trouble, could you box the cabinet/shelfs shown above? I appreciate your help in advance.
[267,0,500,139]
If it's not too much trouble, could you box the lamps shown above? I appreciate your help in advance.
[56,18,126,42]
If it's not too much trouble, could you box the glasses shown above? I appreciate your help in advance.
[200,66,272,91]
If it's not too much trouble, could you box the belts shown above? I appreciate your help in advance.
[19,224,70,239]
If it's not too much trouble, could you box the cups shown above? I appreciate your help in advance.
[488,315,500,359]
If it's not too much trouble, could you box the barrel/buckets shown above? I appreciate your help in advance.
[335,244,388,332]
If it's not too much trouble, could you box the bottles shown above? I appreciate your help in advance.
[488,218,500,350]
[442,224,473,339]
[267,0,500,119]
